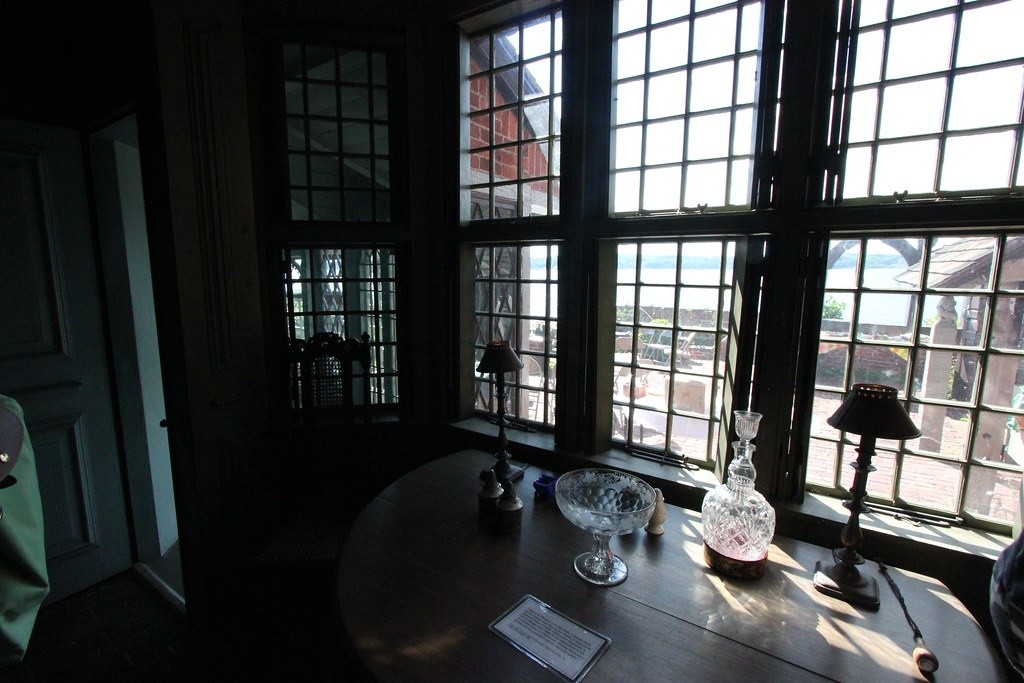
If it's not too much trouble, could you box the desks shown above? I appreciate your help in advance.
[333,446,1010,683]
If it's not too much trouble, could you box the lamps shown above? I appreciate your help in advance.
[478,339,525,484]
[814,384,923,615]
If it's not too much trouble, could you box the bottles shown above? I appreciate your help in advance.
[702,411,776,582]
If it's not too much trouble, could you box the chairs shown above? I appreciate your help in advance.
[282,330,369,522]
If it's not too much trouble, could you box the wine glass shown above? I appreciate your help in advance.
[555,469,657,584]
